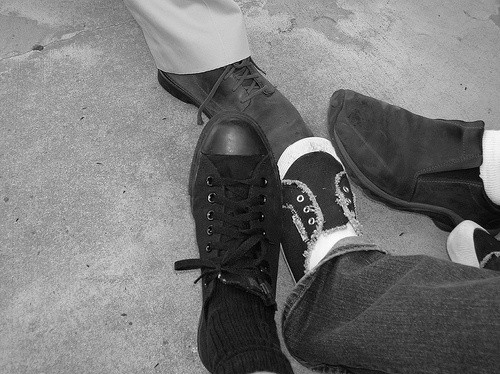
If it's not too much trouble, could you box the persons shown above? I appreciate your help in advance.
[118,0,316,154]
[324,90,500,232]
[186,111,305,373]
[268,135,500,374]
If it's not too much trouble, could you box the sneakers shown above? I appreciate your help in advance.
[273,136,359,286]
[188,113,280,373]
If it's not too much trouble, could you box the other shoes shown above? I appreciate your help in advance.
[447,220,500,277]
[328,88,500,237]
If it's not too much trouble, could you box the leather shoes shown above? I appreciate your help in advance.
[158,57,314,161]
